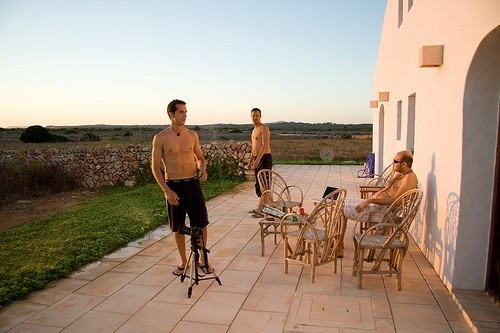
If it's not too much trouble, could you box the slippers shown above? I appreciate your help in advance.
[252,213,264,218]
[198,263,214,274]
[249,210,257,213]
[173,266,190,276]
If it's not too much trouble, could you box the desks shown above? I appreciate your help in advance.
[259,212,308,257]
[310,196,338,222]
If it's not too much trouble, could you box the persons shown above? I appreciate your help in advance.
[151,100,216,276]
[247,108,273,218]
[320,150,419,258]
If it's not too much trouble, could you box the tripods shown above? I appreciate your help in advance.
[180,236,222,298]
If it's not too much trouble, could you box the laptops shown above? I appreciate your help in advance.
[310,186,340,205]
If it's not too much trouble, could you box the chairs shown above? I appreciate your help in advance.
[256,168,303,229]
[360,162,399,198]
[279,187,348,285]
[349,188,426,291]
[359,181,422,234]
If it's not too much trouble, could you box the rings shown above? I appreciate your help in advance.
[205,178,207,179]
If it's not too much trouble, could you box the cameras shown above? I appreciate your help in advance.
[178,225,203,239]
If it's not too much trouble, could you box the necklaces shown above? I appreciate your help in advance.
[169,125,184,136]
[401,170,414,175]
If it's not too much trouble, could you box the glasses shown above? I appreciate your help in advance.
[394,160,407,164]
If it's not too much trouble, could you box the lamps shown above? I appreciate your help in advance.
[418,44,445,68]
[379,91,389,102]
[369,100,378,108]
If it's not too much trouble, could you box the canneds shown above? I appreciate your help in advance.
[281,204,287,214]
[297,207,304,216]
[291,211,297,222]
